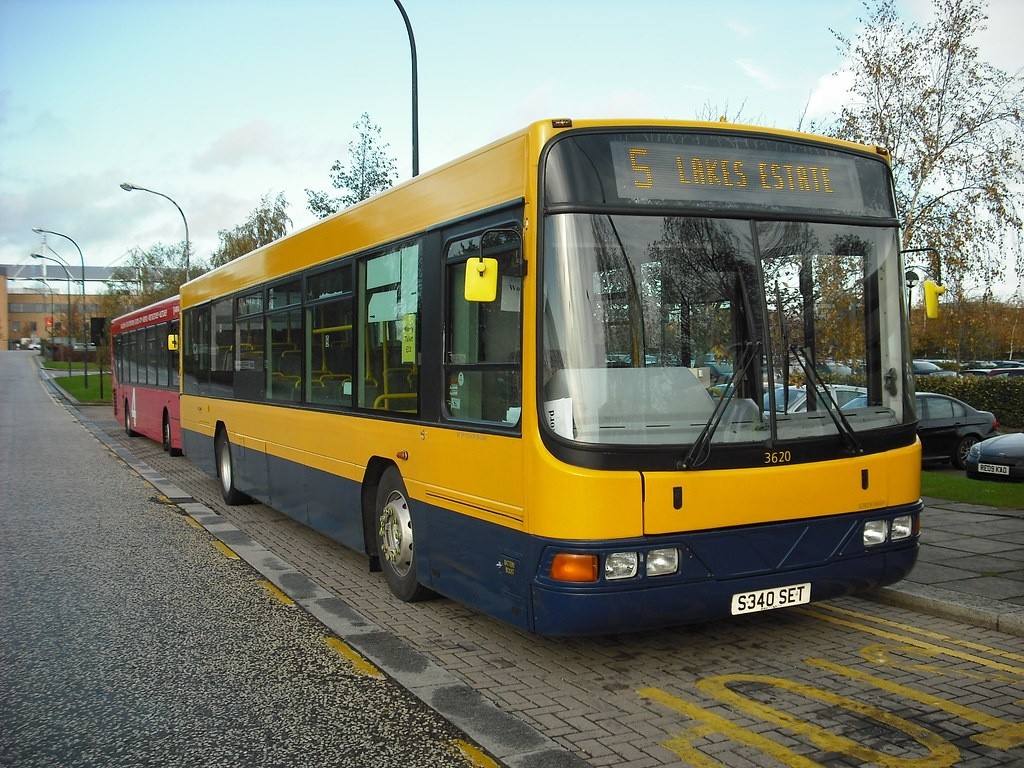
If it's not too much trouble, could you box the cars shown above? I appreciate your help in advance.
[765,356,806,375]
[608,354,656,365]
[816,361,856,374]
[966,431,1024,484]
[711,382,784,398]
[912,360,957,376]
[702,363,733,379]
[837,392,1000,470]
[960,359,1024,376]
[764,384,867,417]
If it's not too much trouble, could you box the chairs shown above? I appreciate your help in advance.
[224,341,417,411]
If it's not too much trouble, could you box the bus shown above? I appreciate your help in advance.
[178,117,947,636]
[109,295,181,457]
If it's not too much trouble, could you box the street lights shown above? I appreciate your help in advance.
[34,228,88,389]
[121,184,190,282]
[904,270,919,342]
[29,277,54,361]
[31,254,71,379]
